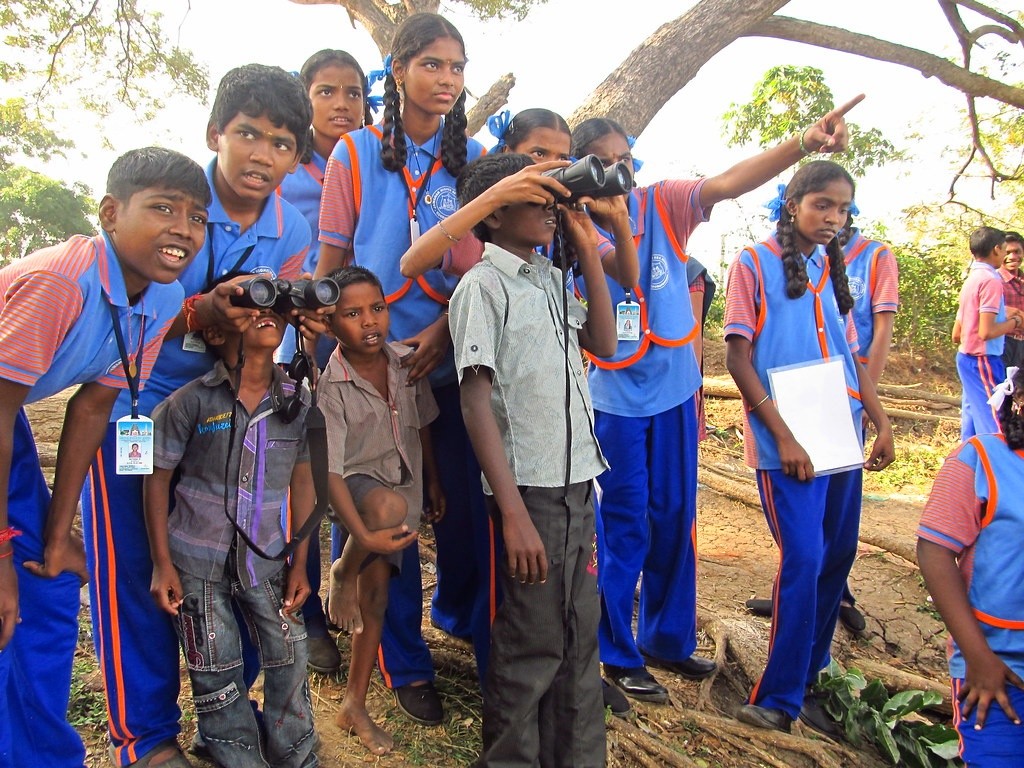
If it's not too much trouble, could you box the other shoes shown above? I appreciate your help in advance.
[394,680,443,725]
[745,599,773,618]
[304,611,341,673]
[600,678,631,717]
[838,603,866,632]
[109,739,193,768]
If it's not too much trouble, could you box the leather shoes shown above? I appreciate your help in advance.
[641,653,717,679]
[603,664,670,703]
[737,704,793,734]
[798,703,843,741]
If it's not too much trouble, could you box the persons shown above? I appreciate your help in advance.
[0,11,1024,768]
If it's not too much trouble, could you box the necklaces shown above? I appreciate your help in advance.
[128,292,145,377]
[407,130,436,204]
[371,385,399,416]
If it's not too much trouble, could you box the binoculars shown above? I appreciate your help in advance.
[231,279,340,310]
[529,154,633,209]
